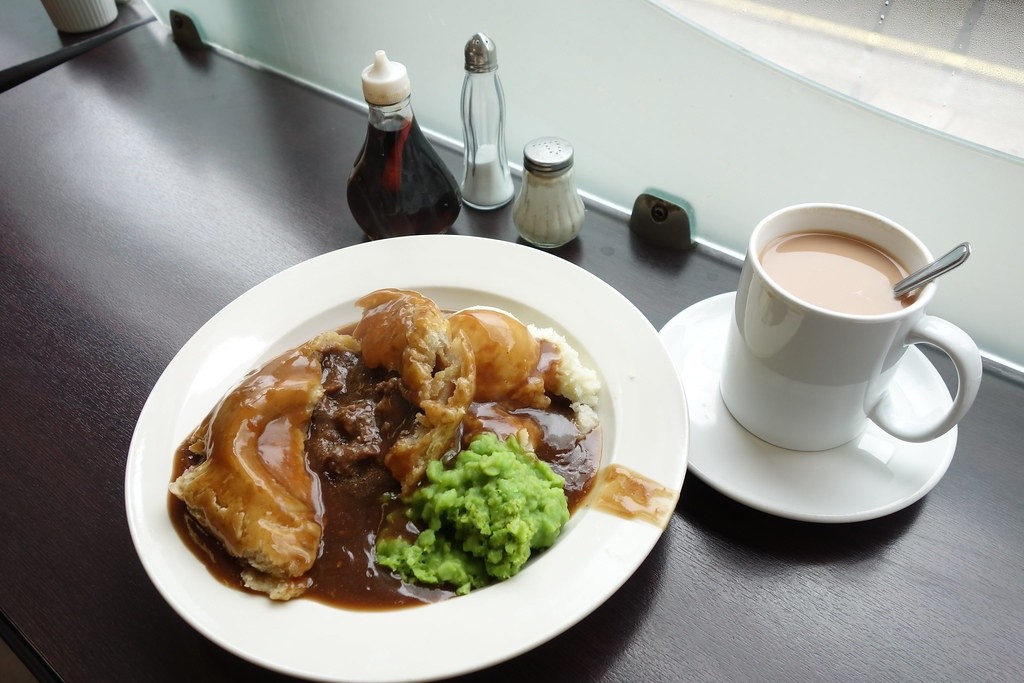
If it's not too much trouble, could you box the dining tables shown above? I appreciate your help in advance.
[0,0,1024,683]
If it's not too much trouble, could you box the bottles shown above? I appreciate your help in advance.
[346,50,461,240]
[460,31,515,211]
[512,136,585,249]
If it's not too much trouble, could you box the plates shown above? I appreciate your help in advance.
[123,234,688,683]
[658,290,959,524]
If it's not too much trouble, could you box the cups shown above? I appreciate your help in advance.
[719,202,983,452]
